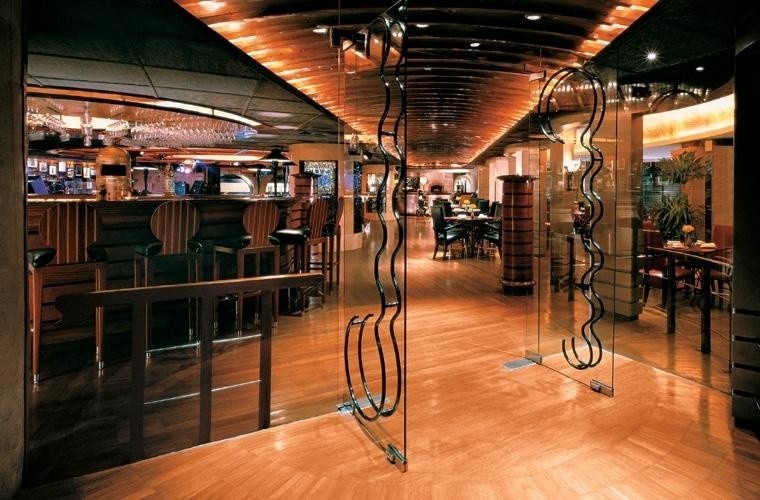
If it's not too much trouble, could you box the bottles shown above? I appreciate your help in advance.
[163,156,188,199]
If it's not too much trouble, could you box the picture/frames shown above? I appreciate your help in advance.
[27,158,97,189]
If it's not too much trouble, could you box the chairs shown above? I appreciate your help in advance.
[430,193,503,261]
[26,196,345,384]
[640,219,734,313]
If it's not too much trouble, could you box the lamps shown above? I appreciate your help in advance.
[248,148,297,196]
[133,162,158,195]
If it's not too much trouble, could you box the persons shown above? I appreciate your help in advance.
[402,179,478,218]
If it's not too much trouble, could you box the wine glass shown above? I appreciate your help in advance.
[24,96,236,147]
